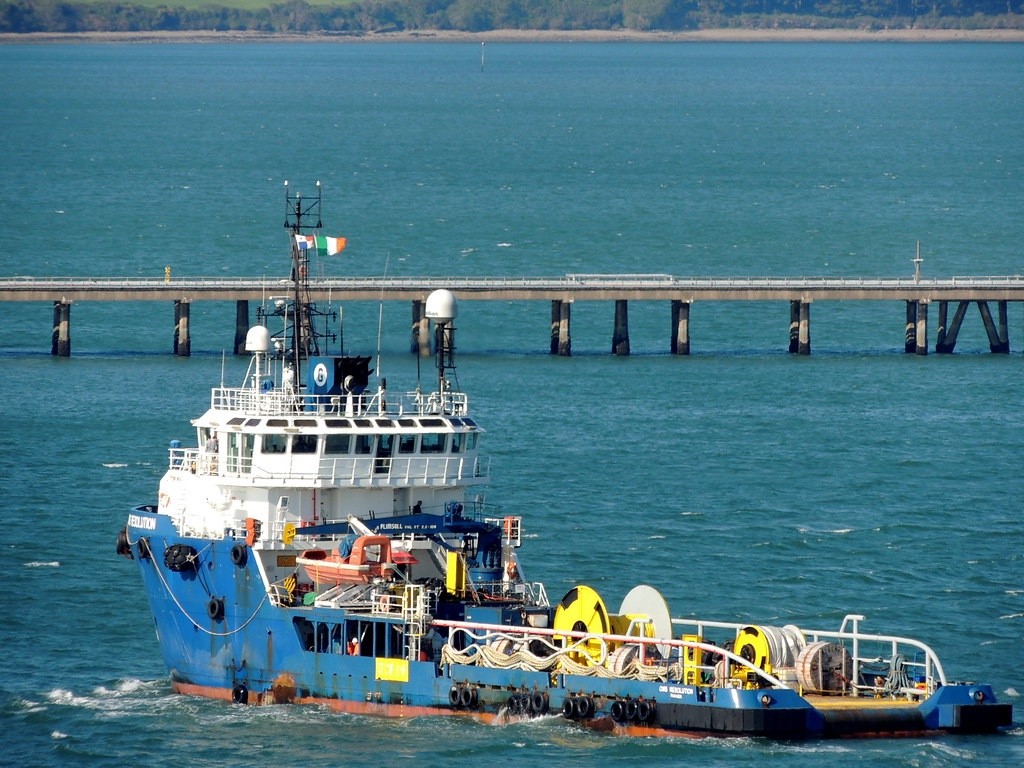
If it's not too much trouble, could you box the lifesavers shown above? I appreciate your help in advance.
[576,696,595,718]
[460,687,478,707]
[230,544,249,566]
[231,685,250,707]
[610,700,656,723]
[507,561,517,580]
[206,598,226,620]
[448,686,462,708]
[507,691,549,716]
[379,595,388,612]
[137,539,151,560]
[562,697,577,720]
[116,532,130,555]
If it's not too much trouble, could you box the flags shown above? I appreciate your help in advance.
[293,233,347,257]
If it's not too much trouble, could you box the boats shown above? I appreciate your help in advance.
[114,177,1015,743]
[296,535,418,585]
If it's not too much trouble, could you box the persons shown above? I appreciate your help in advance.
[320,502,328,525]
[393,499,398,517]
[347,638,360,655]
[413,501,423,513]
[292,435,316,452]
[164,266,172,281]
[377,435,393,449]
[272,444,285,452]
[418,648,427,661]
